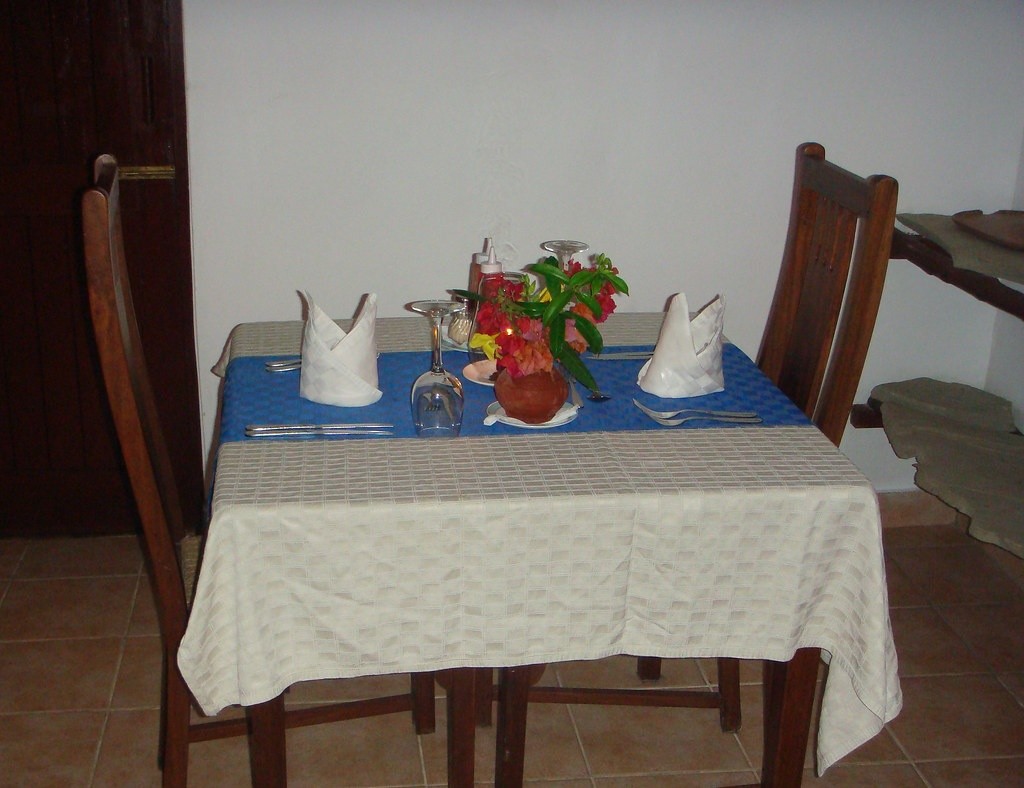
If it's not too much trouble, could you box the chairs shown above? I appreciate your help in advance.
[82,153,475,788]
[494,140,900,787]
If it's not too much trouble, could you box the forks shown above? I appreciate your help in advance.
[632,397,759,419]
[643,410,764,427]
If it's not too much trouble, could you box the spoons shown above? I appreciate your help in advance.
[587,385,611,405]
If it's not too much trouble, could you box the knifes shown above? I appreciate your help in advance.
[586,351,653,360]
[243,422,396,431]
[243,429,395,438]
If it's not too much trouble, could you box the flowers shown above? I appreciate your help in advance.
[449,247,630,395]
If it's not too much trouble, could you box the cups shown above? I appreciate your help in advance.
[448,296,472,344]
[499,271,528,285]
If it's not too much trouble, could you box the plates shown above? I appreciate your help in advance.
[485,399,578,429]
[462,359,499,387]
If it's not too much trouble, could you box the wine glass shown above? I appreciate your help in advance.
[541,241,590,273]
[410,300,467,439]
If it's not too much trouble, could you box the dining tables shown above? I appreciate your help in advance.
[175,313,902,788]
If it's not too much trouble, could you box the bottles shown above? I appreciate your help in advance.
[466,237,506,382]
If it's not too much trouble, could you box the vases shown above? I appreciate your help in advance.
[493,367,569,423]
